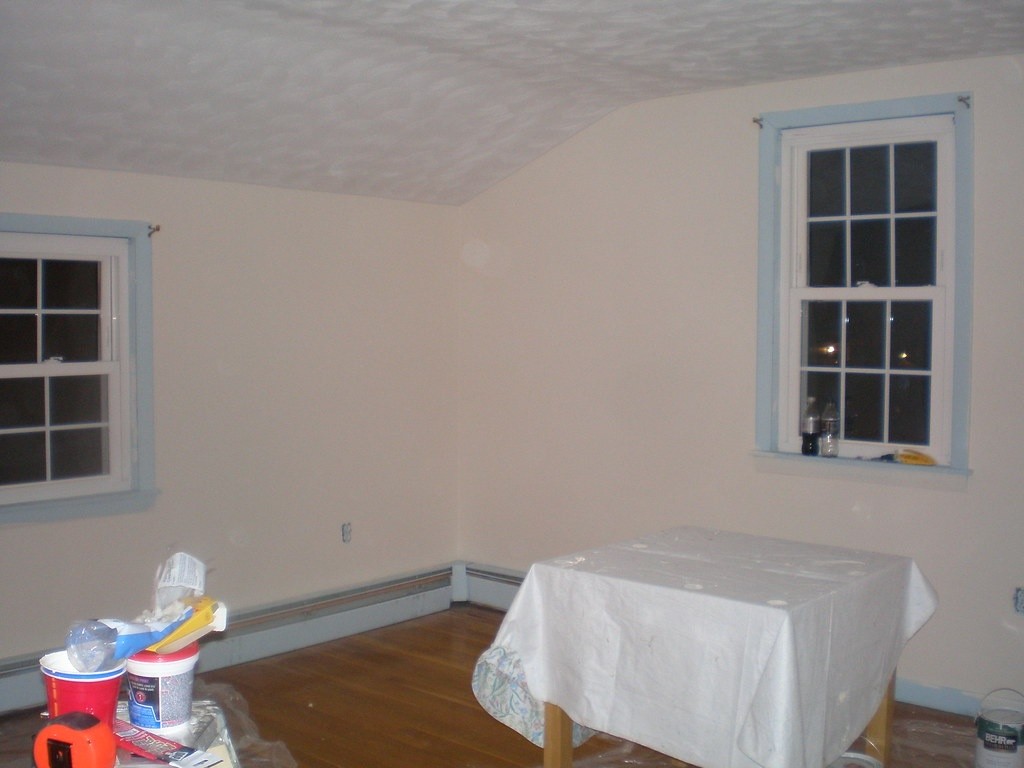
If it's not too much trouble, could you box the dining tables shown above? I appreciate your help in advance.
[469,529,941,768]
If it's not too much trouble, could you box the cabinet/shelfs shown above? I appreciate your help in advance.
[110,699,240,768]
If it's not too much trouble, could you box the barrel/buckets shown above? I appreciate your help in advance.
[38,648,128,733]
[828,751,885,768]
[973,706,1024,767]
[124,636,203,731]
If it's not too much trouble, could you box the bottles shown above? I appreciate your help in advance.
[800,396,821,457]
[821,396,841,458]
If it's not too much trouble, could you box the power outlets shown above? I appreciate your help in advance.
[342,523,351,543]
